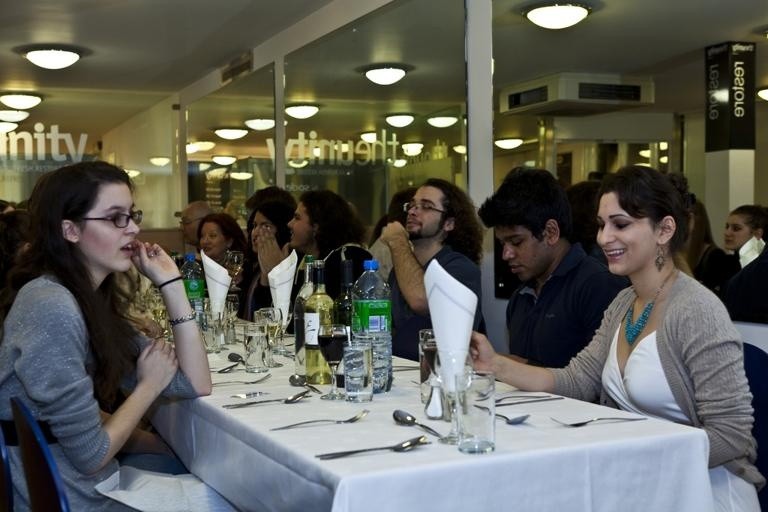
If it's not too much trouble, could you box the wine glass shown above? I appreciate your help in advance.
[317,324,347,402]
[221,294,245,319]
[434,350,478,445]
[257,307,284,370]
[220,251,245,292]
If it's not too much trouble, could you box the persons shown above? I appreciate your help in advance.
[0,165,765,369]
[464,164,766,512]
[0,207,195,478]
[1,160,213,512]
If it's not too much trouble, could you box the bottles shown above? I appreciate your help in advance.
[350,256,392,395]
[292,252,359,385]
[214,312,239,346]
[179,252,204,313]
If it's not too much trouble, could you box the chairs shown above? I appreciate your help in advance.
[739,339,767,512]
[8,393,69,511]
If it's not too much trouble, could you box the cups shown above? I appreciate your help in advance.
[242,324,270,374]
[419,328,442,409]
[343,340,377,399]
[453,370,495,456]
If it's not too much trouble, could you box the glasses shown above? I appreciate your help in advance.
[403,202,446,215]
[179,217,204,226]
[84,209,143,228]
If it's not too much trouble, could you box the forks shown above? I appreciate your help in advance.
[269,408,371,433]
[551,416,649,429]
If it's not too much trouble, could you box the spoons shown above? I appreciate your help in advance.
[389,407,446,442]
[289,371,322,398]
[475,404,527,428]
[227,351,254,371]
[315,432,425,468]
[223,388,310,414]
